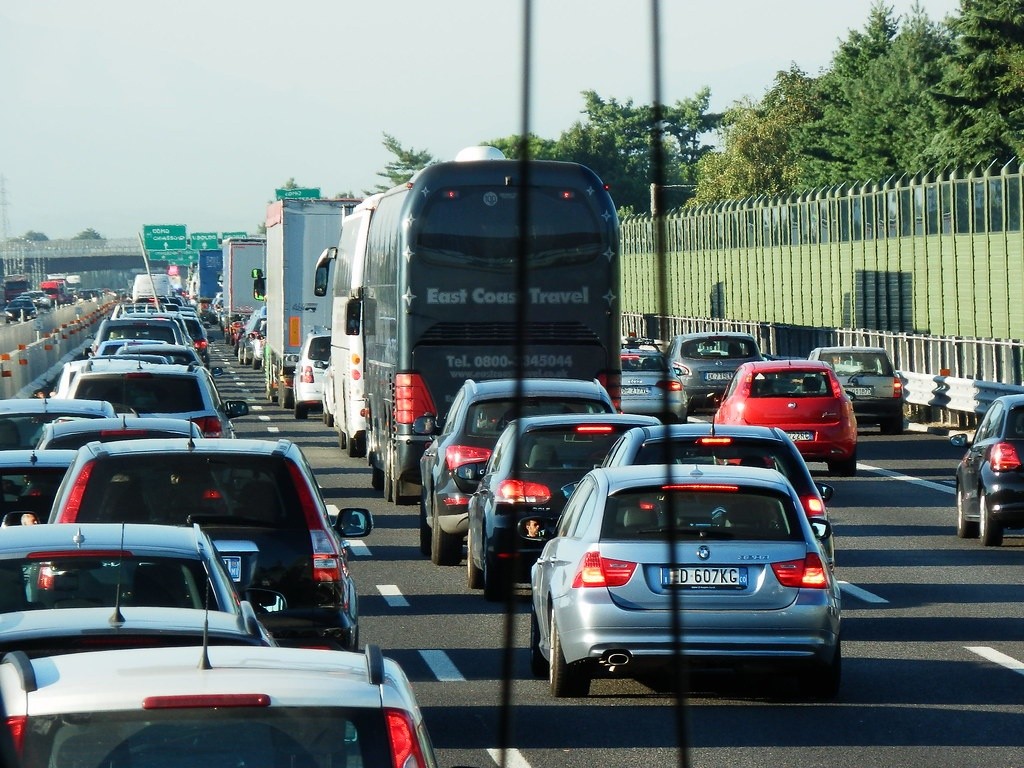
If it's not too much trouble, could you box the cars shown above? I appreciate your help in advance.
[949,394,1024,546]
[4,290,50,324]
[208,292,223,323]
[67,288,111,303]
[0,521,287,663]
[622,347,686,425]
[230,307,266,368]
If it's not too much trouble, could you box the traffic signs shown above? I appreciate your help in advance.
[143,225,246,266]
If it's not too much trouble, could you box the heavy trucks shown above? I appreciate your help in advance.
[217,237,265,339]
[253,198,361,408]
[198,249,223,304]
[41,279,66,306]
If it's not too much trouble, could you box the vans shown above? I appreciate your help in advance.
[133,274,171,305]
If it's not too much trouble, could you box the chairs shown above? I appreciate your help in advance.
[528,444,557,469]
[802,377,821,394]
[641,359,655,369]
[862,360,879,373]
[624,508,658,533]
[729,343,742,358]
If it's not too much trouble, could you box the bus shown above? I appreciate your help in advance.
[316,148,621,504]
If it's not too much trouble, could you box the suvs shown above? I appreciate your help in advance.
[2,437,374,648]
[0,643,438,768]
[416,379,843,704]
[668,331,904,476]
[286,331,331,419]
[0,294,247,534]
